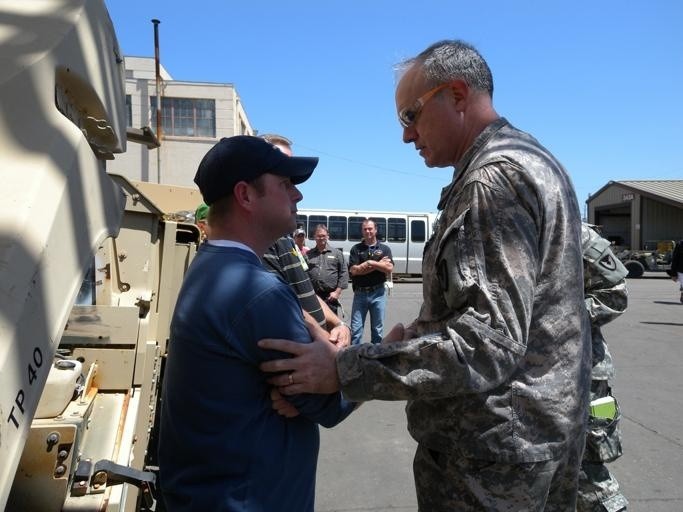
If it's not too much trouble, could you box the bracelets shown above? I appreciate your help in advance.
[366,260,371,266]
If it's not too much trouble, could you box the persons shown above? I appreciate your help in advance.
[148,133,425,512]
[305,224,350,320]
[252,36,599,512]
[559,217,638,512]
[291,229,312,258]
[349,220,395,346]
[666,241,682,303]
[244,132,354,350]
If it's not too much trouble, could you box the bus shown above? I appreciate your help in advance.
[283,208,438,278]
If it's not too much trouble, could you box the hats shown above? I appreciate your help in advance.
[194,136,319,206]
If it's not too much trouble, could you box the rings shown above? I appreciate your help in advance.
[288,373,294,385]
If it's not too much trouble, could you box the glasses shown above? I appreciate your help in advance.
[397,82,448,126]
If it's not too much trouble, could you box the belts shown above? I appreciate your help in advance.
[357,284,383,292]
